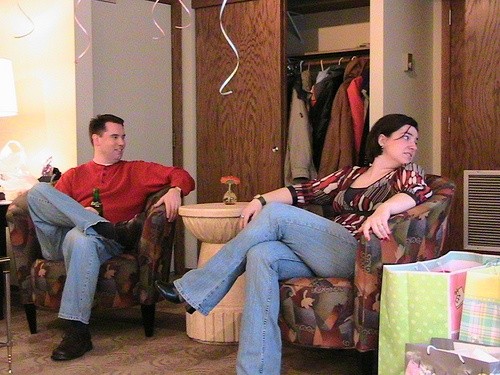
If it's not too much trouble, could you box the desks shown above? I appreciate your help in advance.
[178,203,252,346]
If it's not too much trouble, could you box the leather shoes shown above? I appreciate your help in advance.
[114,213,144,247]
[51,322,93,361]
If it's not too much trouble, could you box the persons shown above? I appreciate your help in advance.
[26,115,195,361]
[154,113,433,375]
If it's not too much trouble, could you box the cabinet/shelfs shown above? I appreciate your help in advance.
[190,0,440,214]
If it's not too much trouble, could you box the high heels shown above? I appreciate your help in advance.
[154,280,197,315]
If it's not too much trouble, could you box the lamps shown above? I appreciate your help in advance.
[0,58,18,117]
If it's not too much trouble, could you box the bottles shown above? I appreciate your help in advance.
[90,189,102,216]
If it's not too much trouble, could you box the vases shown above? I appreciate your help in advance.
[223,191,237,205]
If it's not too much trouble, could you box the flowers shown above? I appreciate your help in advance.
[220,176,240,203]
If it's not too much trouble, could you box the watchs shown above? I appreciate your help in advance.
[171,186,182,191]
[253,194,266,207]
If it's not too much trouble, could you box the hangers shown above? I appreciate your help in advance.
[287,56,364,93]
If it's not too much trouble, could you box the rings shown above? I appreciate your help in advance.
[241,215,245,218]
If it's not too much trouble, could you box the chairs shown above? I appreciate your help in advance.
[280,174,458,352]
[6,173,176,337]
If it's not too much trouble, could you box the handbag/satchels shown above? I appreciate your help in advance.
[378,250,500,375]
[404,337,500,375]
[458,261,500,346]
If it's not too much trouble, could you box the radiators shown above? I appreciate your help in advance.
[463,170,500,252]
